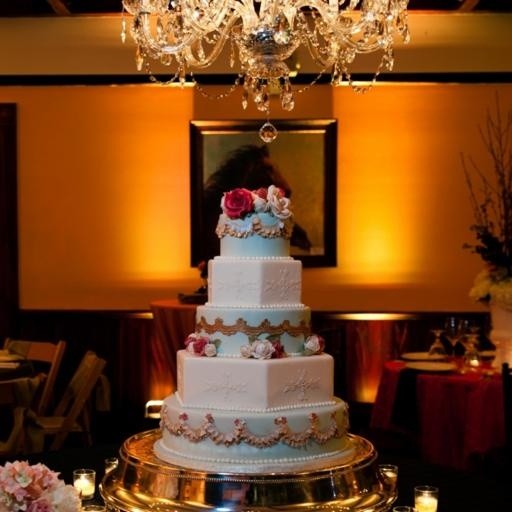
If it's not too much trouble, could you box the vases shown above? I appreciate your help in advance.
[488,303,512,371]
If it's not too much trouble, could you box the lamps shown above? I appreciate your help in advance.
[117,0,415,144]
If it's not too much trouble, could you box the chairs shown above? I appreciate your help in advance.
[0,340,108,466]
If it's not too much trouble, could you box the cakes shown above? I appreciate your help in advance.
[152,185,356,477]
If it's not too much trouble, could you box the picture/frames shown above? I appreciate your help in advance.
[186,120,338,269]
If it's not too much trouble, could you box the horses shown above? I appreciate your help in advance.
[204,144,310,256]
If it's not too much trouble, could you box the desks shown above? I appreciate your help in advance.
[123,298,423,415]
[143,299,210,411]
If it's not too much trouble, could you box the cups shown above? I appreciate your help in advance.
[380,464,399,484]
[104,459,119,473]
[71,468,96,502]
[413,485,441,512]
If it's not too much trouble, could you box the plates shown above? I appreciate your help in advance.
[403,362,457,372]
[402,351,447,360]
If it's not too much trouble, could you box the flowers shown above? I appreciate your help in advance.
[472,268,511,310]
[0,461,82,512]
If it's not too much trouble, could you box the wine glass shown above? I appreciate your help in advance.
[430,314,480,367]
